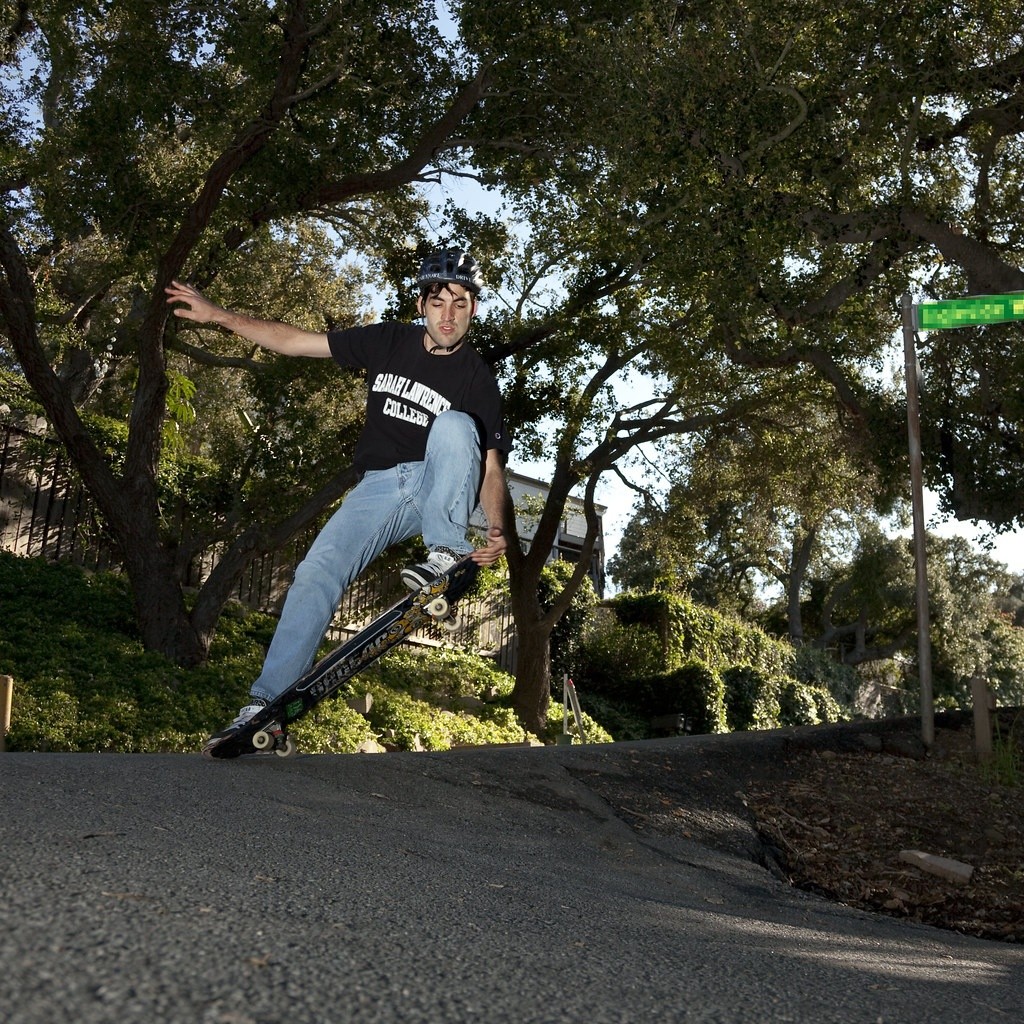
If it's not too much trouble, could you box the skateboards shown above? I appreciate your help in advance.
[206,555,480,761]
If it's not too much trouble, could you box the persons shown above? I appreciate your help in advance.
[163,256,506,756]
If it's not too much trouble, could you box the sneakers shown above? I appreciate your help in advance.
[207,699,267,745]
[401,546,463,592]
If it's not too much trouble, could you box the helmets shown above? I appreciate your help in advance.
[418,250,483,295]
